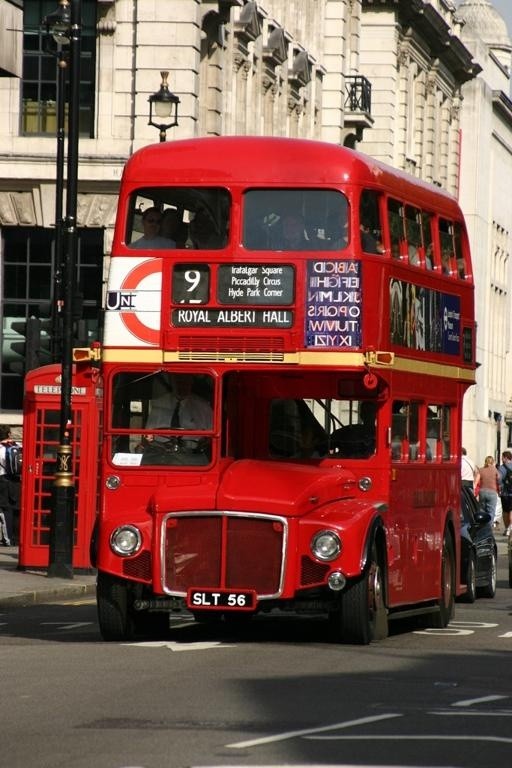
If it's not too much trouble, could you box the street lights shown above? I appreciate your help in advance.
[149,72,179,141]
[40,1,72,363]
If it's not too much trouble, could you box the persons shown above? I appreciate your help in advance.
[474,455,500,527]
[142,374,213,452]
[127,207,176,249]
[0,424,22,546]
[461,447,474,489]
[497,451,512,536]
[282,203,463,279]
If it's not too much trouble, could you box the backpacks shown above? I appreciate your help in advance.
[0,441,23,482]
[501,464,512,494]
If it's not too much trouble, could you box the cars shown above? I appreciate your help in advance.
[460,484,498,605]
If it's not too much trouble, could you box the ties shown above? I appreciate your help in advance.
[171,396,184,439]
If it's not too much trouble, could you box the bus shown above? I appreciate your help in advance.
[92,135,476,646]
